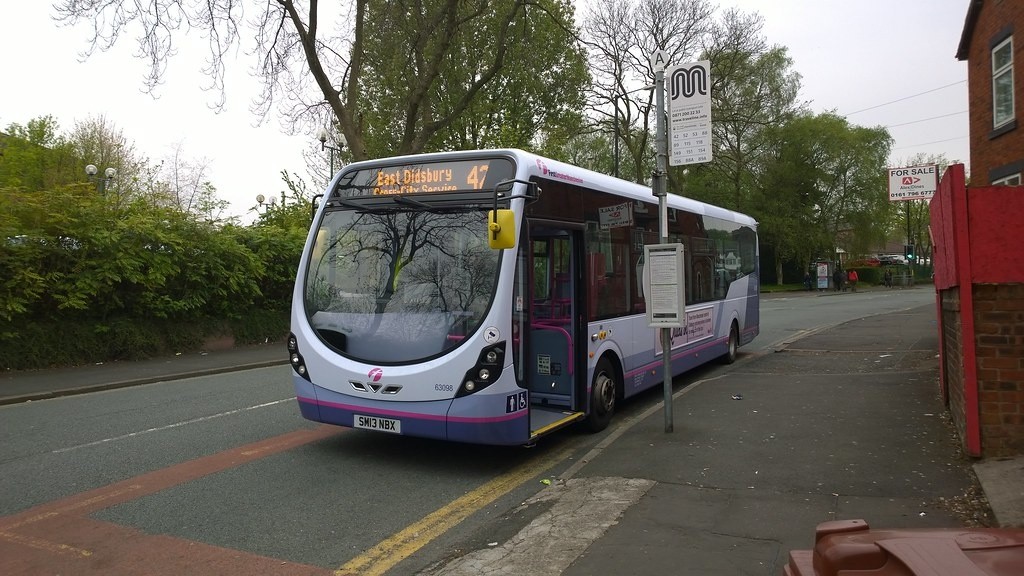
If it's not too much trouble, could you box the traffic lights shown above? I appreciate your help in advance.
[906,245,914,260]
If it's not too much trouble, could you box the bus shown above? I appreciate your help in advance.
[287,147,759,447]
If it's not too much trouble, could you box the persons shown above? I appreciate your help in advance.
[849,268,858,292]
[803,271,813,289]
[834,268,847,292]
[885,268,892,287]
